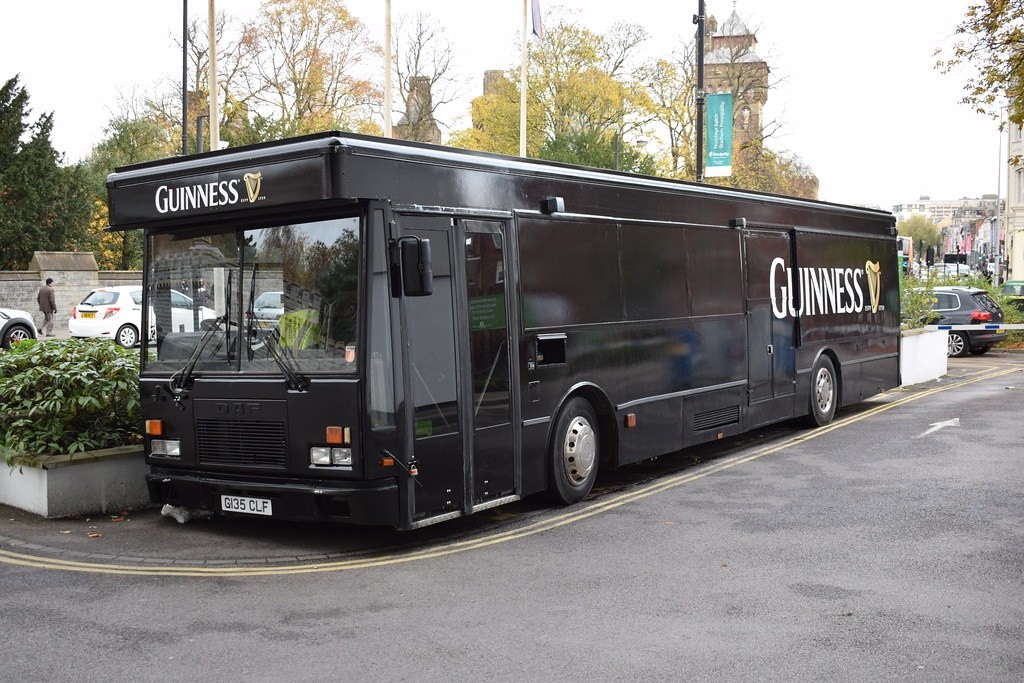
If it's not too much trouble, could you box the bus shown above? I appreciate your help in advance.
[104,125,909,535]
[896,236,914,275]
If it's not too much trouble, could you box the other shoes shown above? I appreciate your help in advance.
[37,329,43,335]
[46,333,56,337]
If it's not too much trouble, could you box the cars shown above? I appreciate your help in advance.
[243,293,284,333]
[1001,281,1024,311]
[0,306,38,355]
[68,285,222,350]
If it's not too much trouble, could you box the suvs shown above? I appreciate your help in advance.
[900,285,1008,358]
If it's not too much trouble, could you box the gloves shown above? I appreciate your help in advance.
[54,309,57,314]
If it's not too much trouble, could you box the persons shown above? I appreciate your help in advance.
[37,278,57,337]
